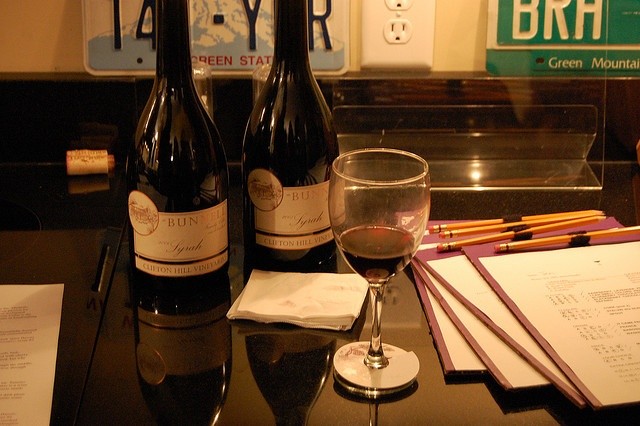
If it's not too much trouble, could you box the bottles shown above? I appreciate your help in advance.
[129,292,232,426]
[242,0,345,285]
[241,336,336,426]
[122,0,230,293]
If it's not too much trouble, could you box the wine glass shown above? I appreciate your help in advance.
[328,149,431,401]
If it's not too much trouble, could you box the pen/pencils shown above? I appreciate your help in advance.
[437,215,609,253]
[440,212,597,240]
[429,209,604,235]
[494,225,639,254]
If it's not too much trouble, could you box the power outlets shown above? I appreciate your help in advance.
[360,1,434,72]
